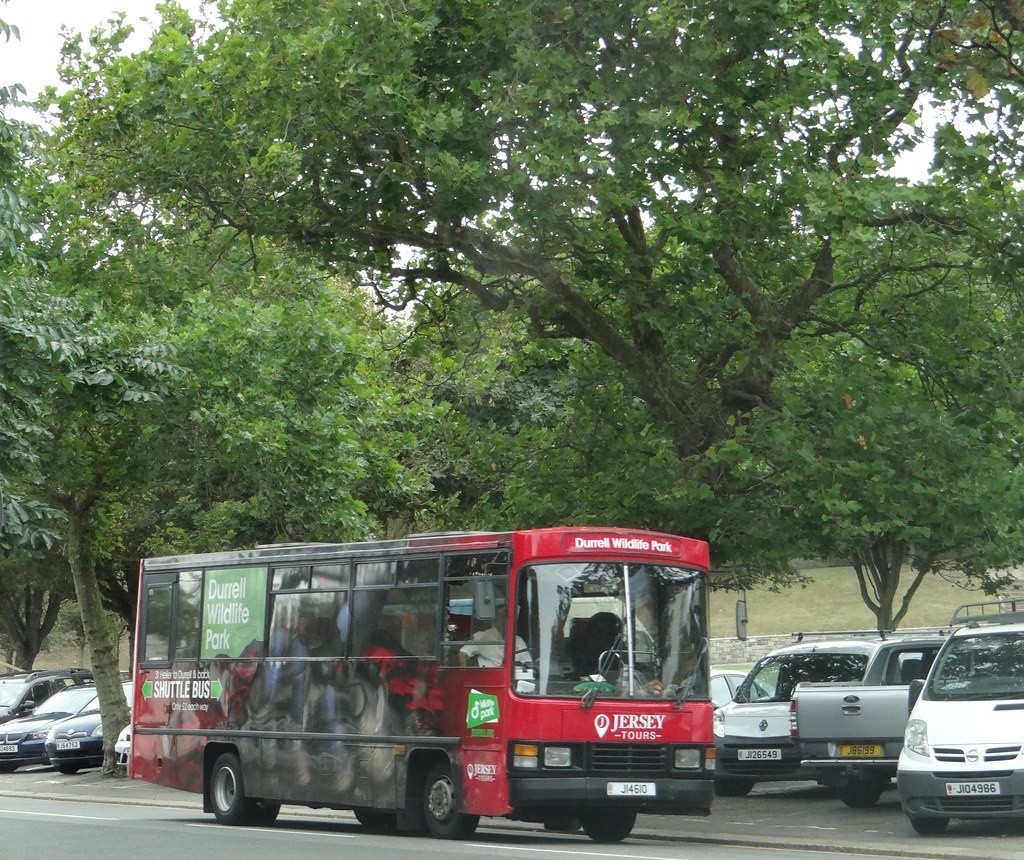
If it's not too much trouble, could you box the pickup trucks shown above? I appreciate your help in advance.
[789,625,971,810]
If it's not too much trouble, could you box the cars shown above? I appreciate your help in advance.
[711,665,776,704]
[0,682,98,772]
[895,597,1024,835]
[45,680,134,775]
[713,628,891,798]
[114,723,131,769]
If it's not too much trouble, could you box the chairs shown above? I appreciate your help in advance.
[400,613,437,657]
[447,613,472,640]
[581,610,624,671]
[901,659,923,682]
[300,616,331,638]
[378,614,401,643]
[114,723,132,777]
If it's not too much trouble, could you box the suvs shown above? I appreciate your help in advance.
[0,668,93,723]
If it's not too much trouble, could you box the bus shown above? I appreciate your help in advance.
[124,525,716,841]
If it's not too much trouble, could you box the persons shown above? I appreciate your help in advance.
[458,604,537,695]
